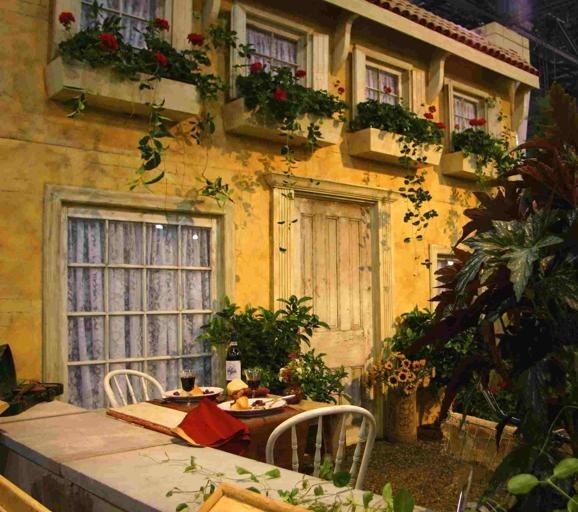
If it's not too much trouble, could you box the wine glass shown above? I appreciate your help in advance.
[179,368,196,396]
[244,367,262,397]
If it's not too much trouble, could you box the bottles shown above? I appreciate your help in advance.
[225,331,244,386]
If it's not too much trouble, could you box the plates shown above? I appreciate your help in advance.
[216,397,286,417]
[163,386,224,403]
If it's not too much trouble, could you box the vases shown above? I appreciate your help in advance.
[283,380,305,405]
[384,390,417,443]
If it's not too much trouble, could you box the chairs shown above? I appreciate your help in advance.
[102,369,162,412]
[266,404,377,491]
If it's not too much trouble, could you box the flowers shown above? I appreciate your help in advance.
[361,348,435,402]
[43,0,235,212]
[225,41,350,255]
[280,350,311,384]
[350,86,446,244]
[444,117,525,190]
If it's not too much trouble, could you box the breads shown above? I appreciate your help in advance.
[227,379,248,392]
[181,386,202,396]
[232,397,252,410]
